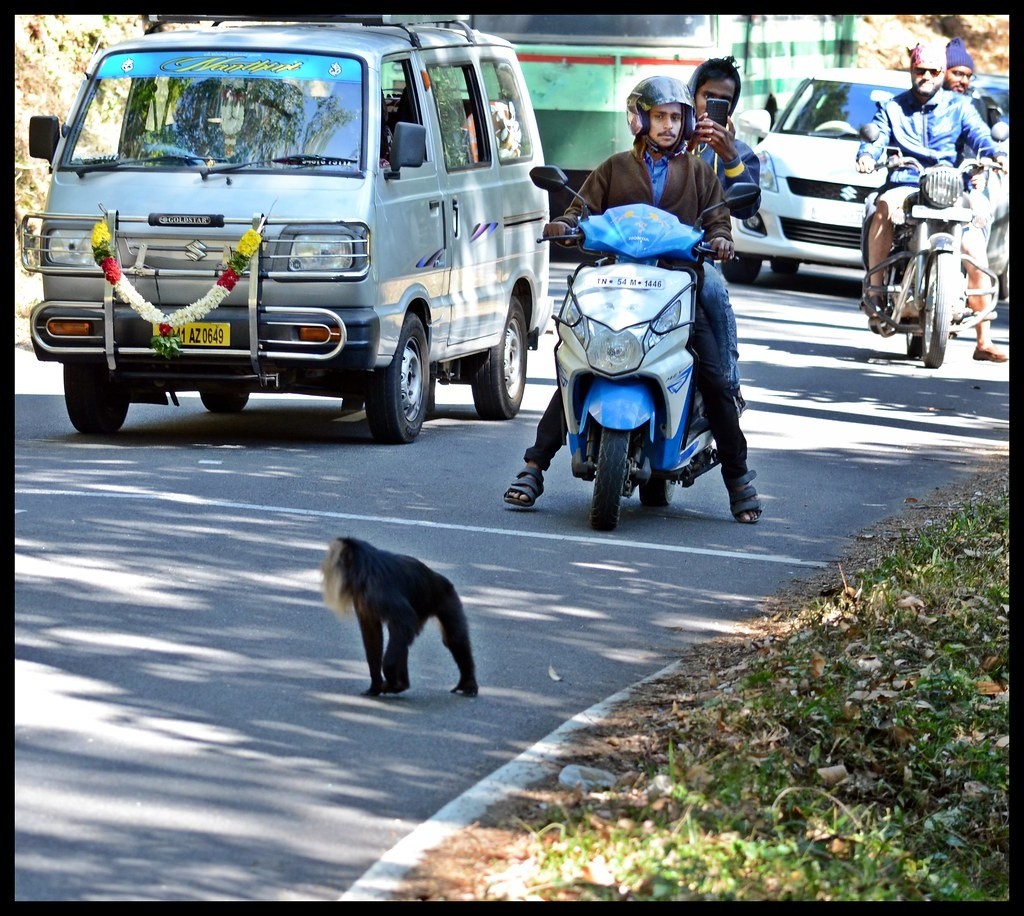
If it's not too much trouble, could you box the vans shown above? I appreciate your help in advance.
[18,18,552,443]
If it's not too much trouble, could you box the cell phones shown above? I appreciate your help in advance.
[706,98,729,128]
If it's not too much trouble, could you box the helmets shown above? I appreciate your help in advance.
[627,76,697,140]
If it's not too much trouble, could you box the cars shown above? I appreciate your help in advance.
[722,69,1007,301]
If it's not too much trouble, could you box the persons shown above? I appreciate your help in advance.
[855,36,1009,362]
[504,55,767,523]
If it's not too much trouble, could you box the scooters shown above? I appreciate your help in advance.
[530,166,761,532]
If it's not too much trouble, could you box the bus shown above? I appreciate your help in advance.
[472,11,862,169]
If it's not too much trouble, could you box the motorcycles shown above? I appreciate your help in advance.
[861,119,1009,369]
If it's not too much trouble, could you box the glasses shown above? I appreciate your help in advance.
[912,67,942,79]
[949,70,972,77]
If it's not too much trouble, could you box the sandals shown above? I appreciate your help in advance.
[504,466,544,507]
[721,466,761,523]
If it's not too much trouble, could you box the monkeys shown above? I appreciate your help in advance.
[322,537,479,699]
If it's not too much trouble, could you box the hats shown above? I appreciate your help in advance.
[945,37,975,72]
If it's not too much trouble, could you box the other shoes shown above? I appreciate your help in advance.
[864,295,885,318]
[973,347,1008,363]
